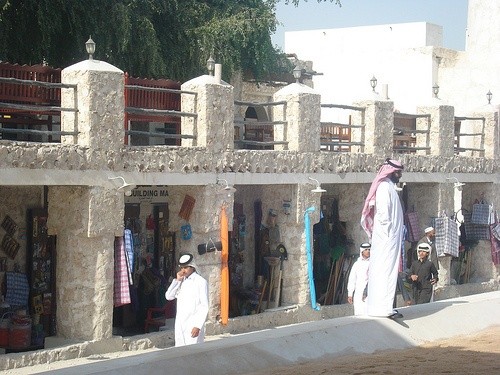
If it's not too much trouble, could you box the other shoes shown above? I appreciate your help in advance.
[389,309,404,319]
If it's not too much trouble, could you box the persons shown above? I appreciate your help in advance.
[393,227,438,310]
[347,243,371,316]
[361,158,406,319]
[164,254,209,347]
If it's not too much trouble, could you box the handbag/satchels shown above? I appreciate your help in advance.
[463,197,500,266]
[433,210,460,258]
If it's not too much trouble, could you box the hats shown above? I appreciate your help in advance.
[425,226,433,233]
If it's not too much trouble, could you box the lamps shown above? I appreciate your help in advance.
[446,175,466,192]
[486,90,493,104]
[370,75,378,93]
[293,65,301,83]
[216,177,237,198]
[433,82,439,96]
[86,34,96,59]
[308,176,329,194]
[206,51,215,76]
[109,176,135,197]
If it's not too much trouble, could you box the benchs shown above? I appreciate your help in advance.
[0,62,62,142]
[124,72,181,146]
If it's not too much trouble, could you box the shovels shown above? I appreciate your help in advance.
[275,244,287,306]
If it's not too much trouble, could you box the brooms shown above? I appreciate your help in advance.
[263,252,282,309]
[323,244,347,306]
